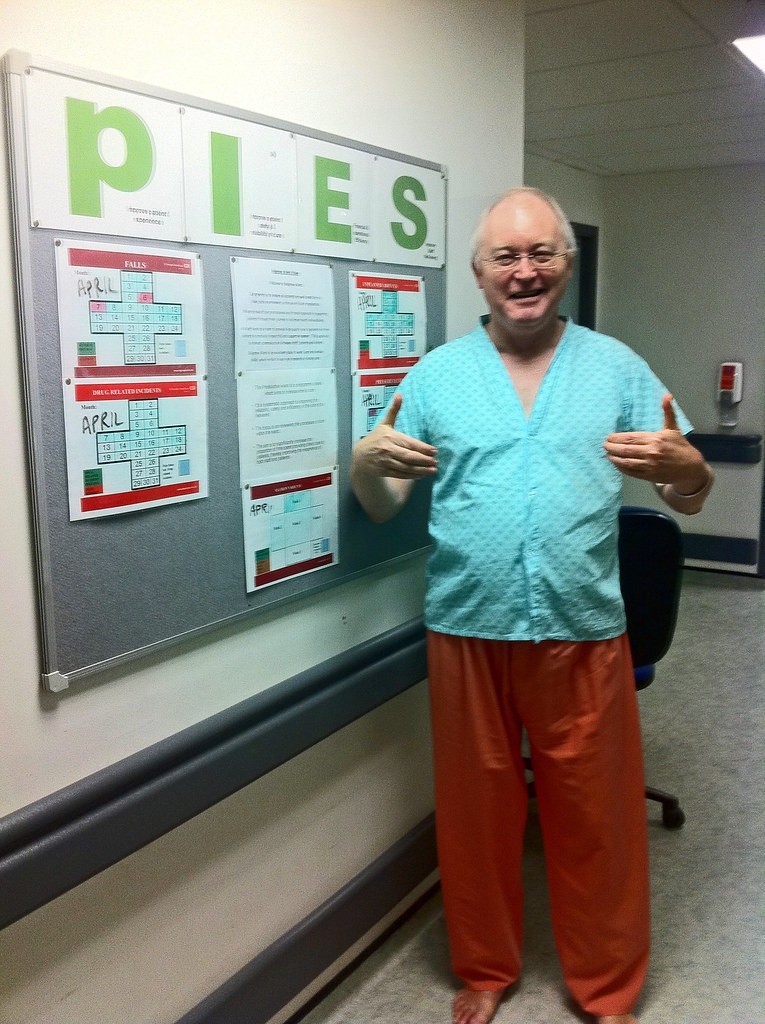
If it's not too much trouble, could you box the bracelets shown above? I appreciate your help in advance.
[671,476,711,499]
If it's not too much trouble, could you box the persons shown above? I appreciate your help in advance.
[350,187,714,1024]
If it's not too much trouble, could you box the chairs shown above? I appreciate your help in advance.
[515,507,688,830]
